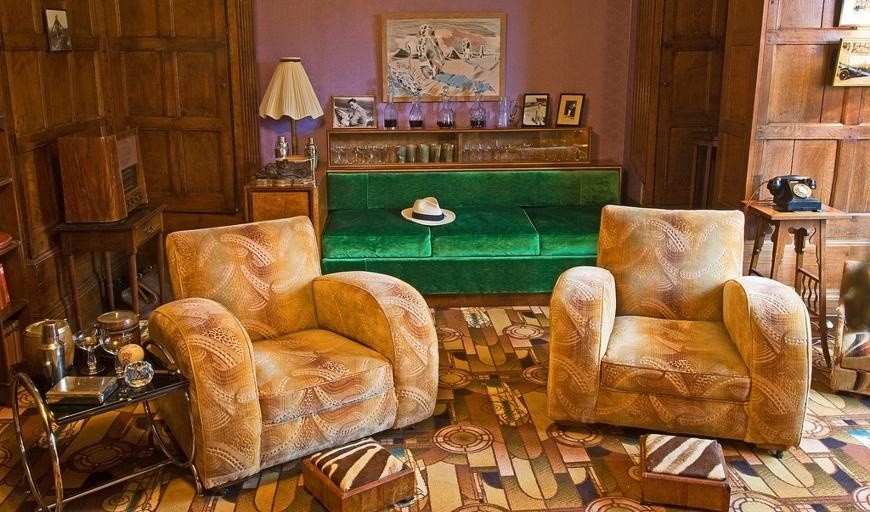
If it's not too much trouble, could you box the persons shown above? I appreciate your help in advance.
[347,99,367,127]
[535,103,545,125]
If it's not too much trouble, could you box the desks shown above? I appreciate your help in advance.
[52,204,169,332]
[10,344,197,512]
[739,199,852,372]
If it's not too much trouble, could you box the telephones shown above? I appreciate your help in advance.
[767,175,821,213]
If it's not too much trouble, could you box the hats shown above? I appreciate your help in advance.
[401,197,456,226]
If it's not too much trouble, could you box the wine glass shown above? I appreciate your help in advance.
[99,335,130,379]
[332,143,398,165]
[71,328,107,375]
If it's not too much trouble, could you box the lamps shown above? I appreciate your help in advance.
[259,56,324,163]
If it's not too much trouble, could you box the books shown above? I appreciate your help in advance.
[1,263,23,382]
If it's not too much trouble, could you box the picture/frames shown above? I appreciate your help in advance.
[377,12,508,102]
[44,7,73,54]
[330,94,378,130]
[522,94,550,126]
[555,93,586,125]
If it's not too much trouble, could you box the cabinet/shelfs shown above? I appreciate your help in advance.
[243,163,327,256]
[326,101,592,167]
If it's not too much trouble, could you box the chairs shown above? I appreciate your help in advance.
[142,215,438,499]
[547,203,813,459]
[826,261,870,395]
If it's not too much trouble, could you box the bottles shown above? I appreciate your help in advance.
[39,320,66,386]
[274,137,289,158]
[383,92,398,131]
[409,93,424,130]
[469,92,487,128]
[304,137,318,169]
[437,95,455,127]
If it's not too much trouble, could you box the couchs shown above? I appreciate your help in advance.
[320,161,625,294]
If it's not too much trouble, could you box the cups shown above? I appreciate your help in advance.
[397,146,407,163]
[419,143,429,162]
[443,143,455,162]
[430,143,441,162]
[495,96,520,128]
[406,144,418,162]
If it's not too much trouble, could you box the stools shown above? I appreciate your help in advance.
[687,136,720,208]
[638,433,731,511]
[301,435,420,510]
